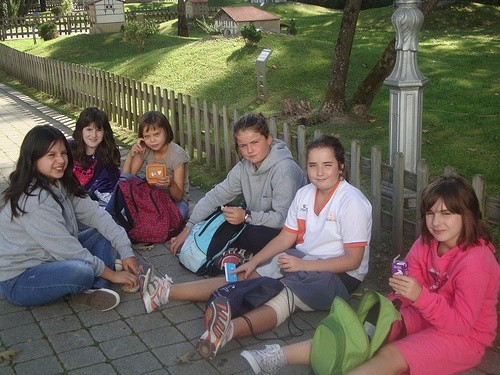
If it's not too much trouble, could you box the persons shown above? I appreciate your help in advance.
[135,135,374,361]
[169,112,310,273]
[66,107,122,209]
[0,122,144,312]
[105,110,191,244]
[240,173,500,375]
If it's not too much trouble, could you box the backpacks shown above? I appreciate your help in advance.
[310,291,402,375]
[115,177,183,243]
[177,210,246,273]
[205,276,287,319]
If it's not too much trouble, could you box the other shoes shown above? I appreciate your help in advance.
[72,288,120,312]
[214,249,254,270]
[113,259,123,272]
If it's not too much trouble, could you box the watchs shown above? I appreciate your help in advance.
[243,210,253,224]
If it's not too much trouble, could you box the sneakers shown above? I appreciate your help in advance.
[135,262,170,312]
[196,296,233,360]
[240,343,290,374]
